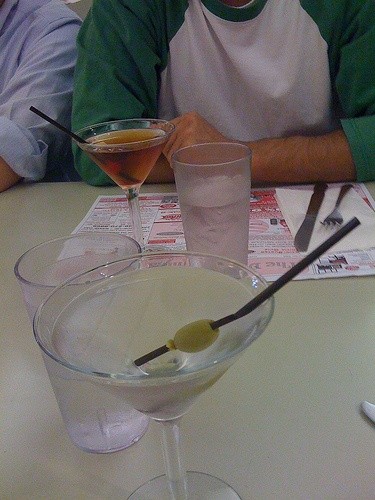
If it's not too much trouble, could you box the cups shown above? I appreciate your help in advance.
[14,232,150,453]
[172,142,252,279]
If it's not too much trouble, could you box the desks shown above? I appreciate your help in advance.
[0,182,375,500]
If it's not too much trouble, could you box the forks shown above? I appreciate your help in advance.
[319,184,352,225]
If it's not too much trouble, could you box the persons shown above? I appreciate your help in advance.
[70,0,375,187]
[0,0,84,193]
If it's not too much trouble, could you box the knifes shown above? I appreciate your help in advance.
[293,182,328,252]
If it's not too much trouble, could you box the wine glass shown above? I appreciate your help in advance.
[73,118,176,254]
[33,252,275,500]
